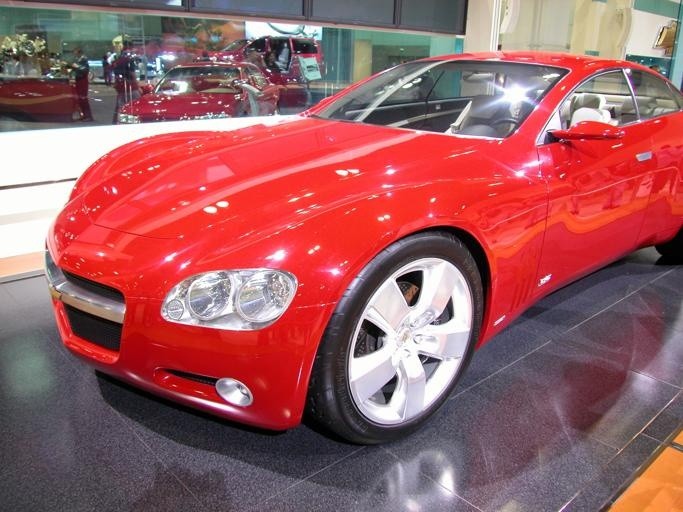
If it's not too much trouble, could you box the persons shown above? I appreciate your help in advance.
[102,40,146,125]
[265,51,280,115]
[0,48,93,122]
[189,50,209,76]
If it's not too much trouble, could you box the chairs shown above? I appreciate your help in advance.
[458,93,669,140]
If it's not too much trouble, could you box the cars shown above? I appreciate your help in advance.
[0,52,116,123]
[117,38,319,127]
[43,49,683,447]
